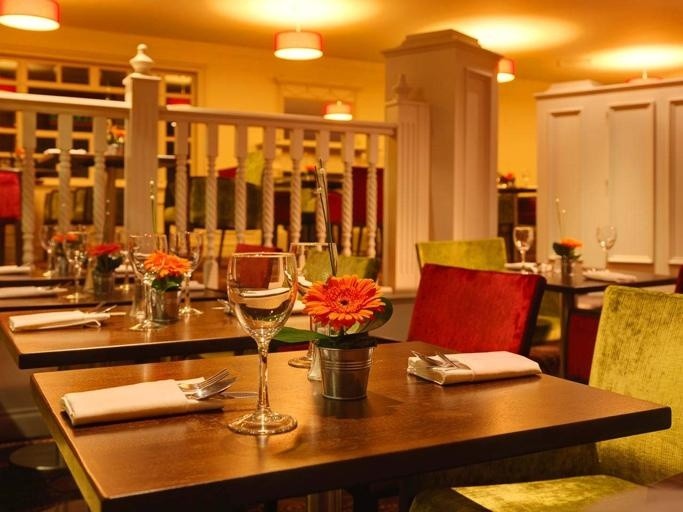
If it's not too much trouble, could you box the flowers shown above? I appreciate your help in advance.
[551,194,580,259]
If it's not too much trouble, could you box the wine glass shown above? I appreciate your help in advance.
[286,238,337,372]
[178,233,205,318]
[36,223,62,277]
[512,226,534,274]
[64,233,91,298]
[127,231,167,332]
[226,250,301,438]
[595,224,617,273]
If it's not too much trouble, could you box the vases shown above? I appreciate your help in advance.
[317,337,373,400]
[558,253,575,281]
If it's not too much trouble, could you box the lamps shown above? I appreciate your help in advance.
[0,1,64,35]
[268,1,327,64]
[320,97,354,122]
[495,53,517,86]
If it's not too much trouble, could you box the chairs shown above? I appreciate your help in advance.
[0,145,542,258]
[405,259,548,357]
[564,259,683,379]
[409,235,565,343]
[434,278,683,511]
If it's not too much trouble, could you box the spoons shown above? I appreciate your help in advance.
[190,377,236,401]
[408,349,442,369]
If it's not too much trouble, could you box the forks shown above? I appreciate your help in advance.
[182,366,228,391]
[434,352,460,370]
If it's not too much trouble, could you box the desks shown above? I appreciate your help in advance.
[25,335,674,512]
[0,259,385,369]
[499,263,683,379]
